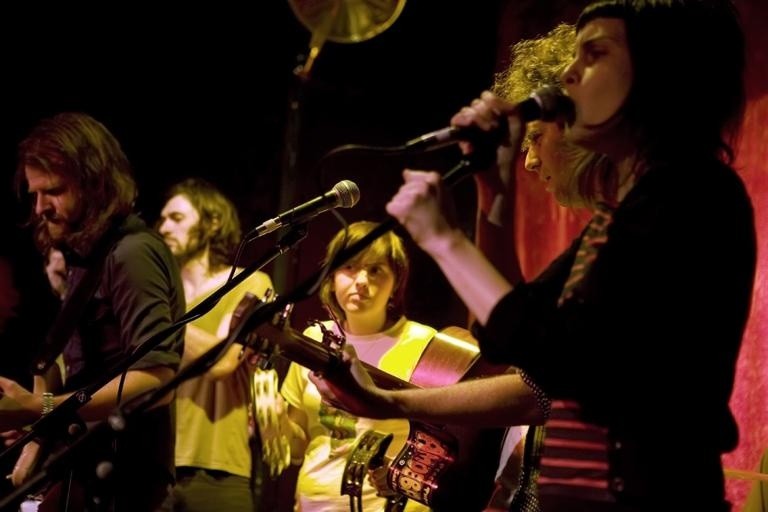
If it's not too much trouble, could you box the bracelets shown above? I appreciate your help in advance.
[41,391,53,418]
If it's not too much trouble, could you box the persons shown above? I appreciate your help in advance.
[0,178,447,512]
[0,112,184,510]
[321,19,601,512]
[385,1,762,511]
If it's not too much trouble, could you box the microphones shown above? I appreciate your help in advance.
[255,180,361,239]
[420,85,565,153]
[440,147,498,187]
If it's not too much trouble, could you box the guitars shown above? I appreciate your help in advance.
[1,364,63,487]
[228,290,515,510]
